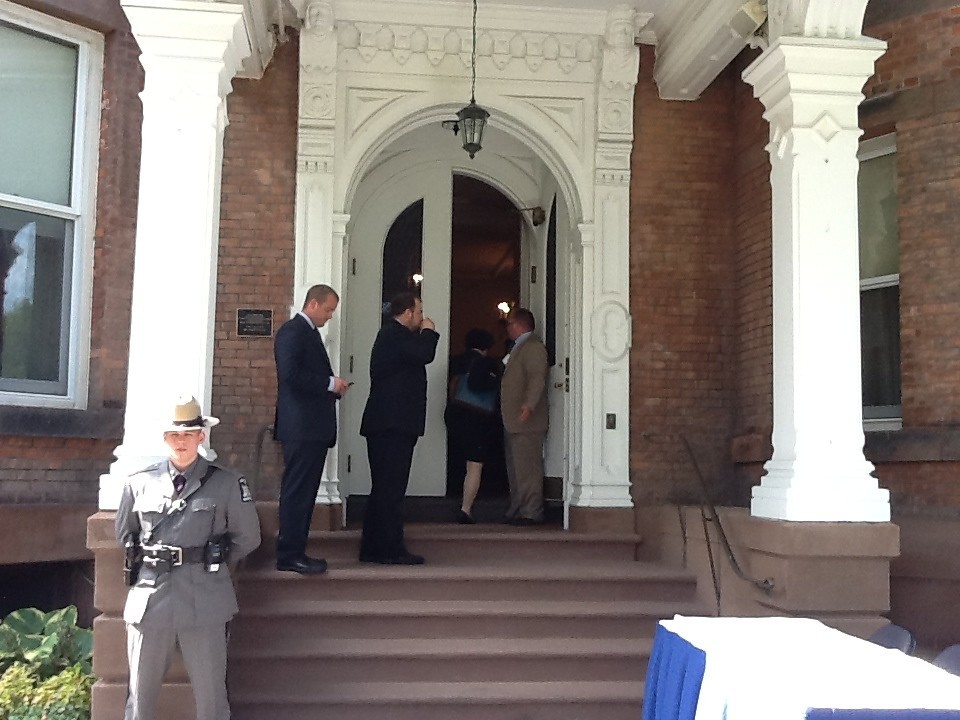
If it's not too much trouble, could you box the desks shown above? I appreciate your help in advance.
[642,617,960,720]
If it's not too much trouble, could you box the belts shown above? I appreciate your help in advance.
[137,546,228,566]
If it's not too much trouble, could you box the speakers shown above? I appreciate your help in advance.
[728,2,767,38]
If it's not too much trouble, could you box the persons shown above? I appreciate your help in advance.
[359,293,440,564]
[115,396,262,720]
[272,285,350,574]
[445,327,502,524]
[489,307,549,525]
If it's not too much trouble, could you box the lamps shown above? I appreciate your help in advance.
[455,0,490,160]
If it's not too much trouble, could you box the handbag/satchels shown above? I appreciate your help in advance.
[453,356,501,414]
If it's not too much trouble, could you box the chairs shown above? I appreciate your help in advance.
[931,644,960,676]
[868,624,916,655]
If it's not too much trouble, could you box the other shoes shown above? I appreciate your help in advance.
[457,510,474,523]
[510,518,545,526]
[360,550,424,564]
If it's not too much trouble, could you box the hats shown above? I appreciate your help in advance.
[155,395,220,430]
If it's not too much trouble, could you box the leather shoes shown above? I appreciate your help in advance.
[276,553,327,573]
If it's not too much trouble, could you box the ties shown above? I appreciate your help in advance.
[173,474,184,495]
